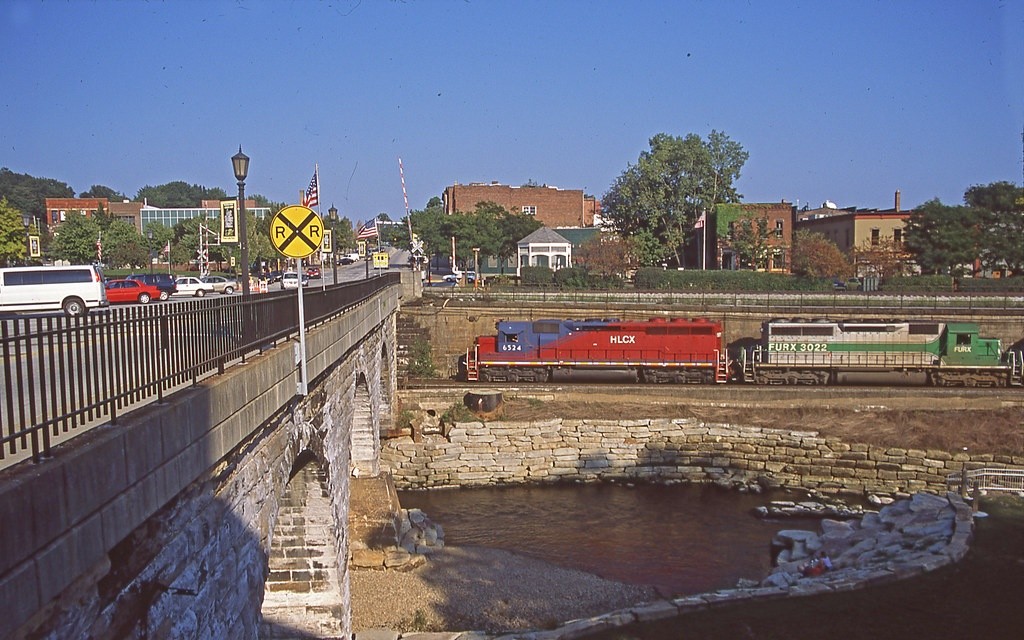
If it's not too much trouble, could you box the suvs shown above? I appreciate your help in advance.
[442,271,476,282]
[844,277,862,286]
[280,272,298,290]
[0,265,108,318]
[237,273,254,287]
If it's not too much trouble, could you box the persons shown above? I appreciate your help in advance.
[421,268,426,282]
[819,551,832,571]
[798,560,825,577]
[303,259,307,270]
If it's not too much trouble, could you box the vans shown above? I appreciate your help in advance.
[124,274,180,302]
[334,253,360,266]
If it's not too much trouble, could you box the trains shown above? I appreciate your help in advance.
[461,318,1024,388]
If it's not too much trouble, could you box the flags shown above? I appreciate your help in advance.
[163,242,168,252]
[357,218,377,239]
[304,172,318,208]
[97,239,102,259]
[695,211,704,228]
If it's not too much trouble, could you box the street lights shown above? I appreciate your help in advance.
[147,228,153,274]
[365,237,370,281]
[328,203,339,285]
[473,247,480,296]
[230,143,250,338]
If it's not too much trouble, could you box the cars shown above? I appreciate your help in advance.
[302,273,309,288]
[104,280,162,304]
[365,247,386,261]
[258,270,285,284]
[307,268,321,279]
[170,277,215,297]
[200,276,238,295]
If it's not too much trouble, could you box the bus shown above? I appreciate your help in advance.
[373,253,391,269]
